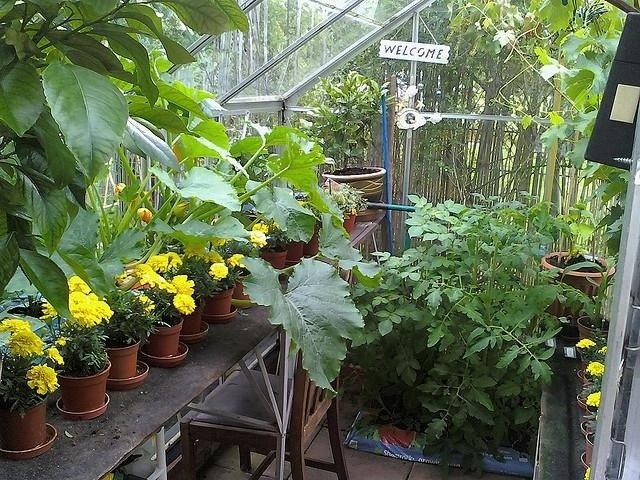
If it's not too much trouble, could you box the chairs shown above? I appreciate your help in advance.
[180,347,349,480]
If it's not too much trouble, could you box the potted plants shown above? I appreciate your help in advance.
[300,69,386,222]
[542,203,616,322]
[561,257,610,341]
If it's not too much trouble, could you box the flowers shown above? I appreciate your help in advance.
[576,338,608,480]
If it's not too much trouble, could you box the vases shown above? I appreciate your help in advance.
[0,395,57,460]
